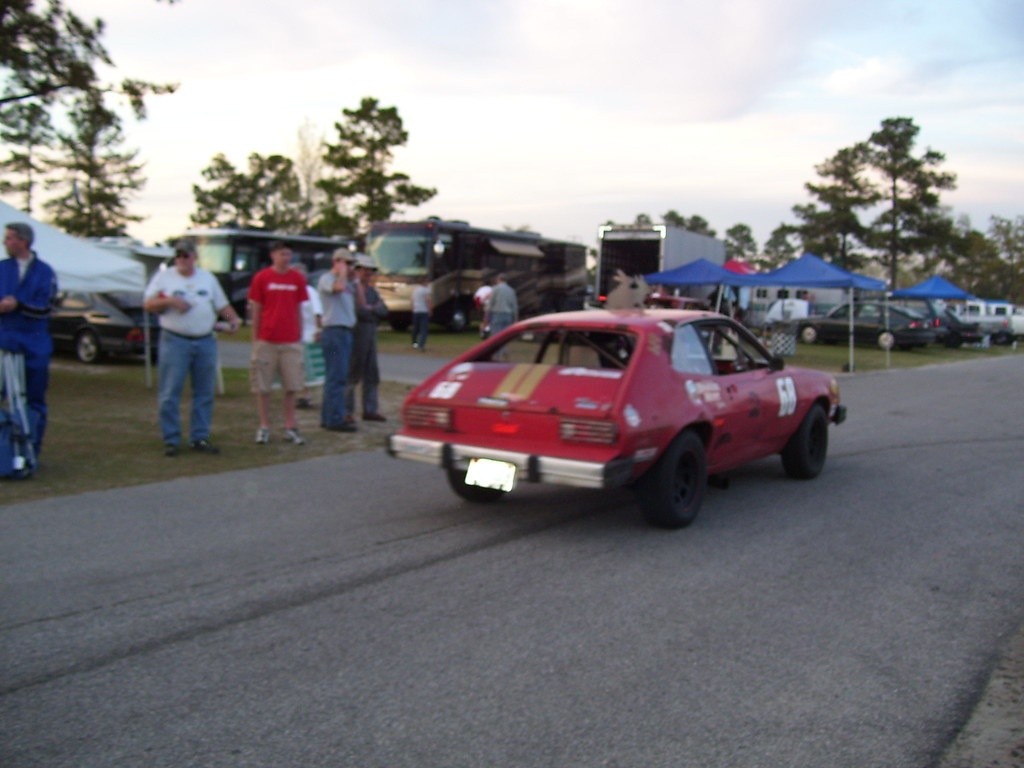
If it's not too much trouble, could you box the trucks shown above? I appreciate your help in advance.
[597,224,728,319]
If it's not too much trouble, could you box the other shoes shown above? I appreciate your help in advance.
[256,428,269,444]
[165,445,175,456]
[324,423,357,432]
[362,411,386,421]
[192,439,219,454]
[344,415,353,424]
[282,429,305,445]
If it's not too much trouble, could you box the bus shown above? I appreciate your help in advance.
[360,214,588,334]
[166,229,355,323]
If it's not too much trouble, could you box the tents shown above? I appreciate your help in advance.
[0,200,153,388]
[644,252,890,372]
[894,275,975,301]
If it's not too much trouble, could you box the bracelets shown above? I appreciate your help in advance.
[353,279,359,283]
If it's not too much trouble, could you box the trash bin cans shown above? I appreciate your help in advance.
[772,319,799,357]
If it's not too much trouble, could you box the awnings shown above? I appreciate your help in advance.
[488,237,544,258]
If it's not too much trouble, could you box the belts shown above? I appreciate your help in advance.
[162,328,212,340]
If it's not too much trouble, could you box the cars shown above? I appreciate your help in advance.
[381,306,848,529]
[914,297,1024,349]
[794,297,936,351]
[47,286,159,364]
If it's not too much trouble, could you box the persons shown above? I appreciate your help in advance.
[346,253,388,424]
[143,242,240,456]
[707,286,723,312]
[410,275,433,353]
[247,241,310,445]
[296,284,324,410]
[0,224,58,457]
[317,248,366,433]
[475,273,519,361]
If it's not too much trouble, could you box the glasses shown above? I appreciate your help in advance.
[177,253,190,259]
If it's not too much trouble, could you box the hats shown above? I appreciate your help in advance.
[333,248,359,263]
[348,255,378,275]
[177,241,195,253]
